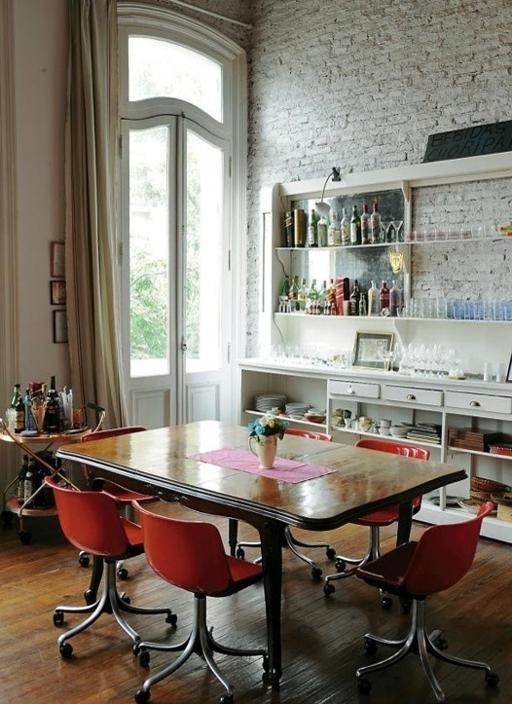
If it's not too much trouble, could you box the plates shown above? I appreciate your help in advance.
[255,392,287,413]
[284,400,309,418]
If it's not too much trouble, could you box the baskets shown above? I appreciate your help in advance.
[471,476,511,504]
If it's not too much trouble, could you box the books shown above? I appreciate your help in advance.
[406,424,441,445]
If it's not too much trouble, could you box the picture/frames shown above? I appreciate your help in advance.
[54,310,67,343]
[51,241,65,278]
[51,280,66,305]
[352,330,395,371]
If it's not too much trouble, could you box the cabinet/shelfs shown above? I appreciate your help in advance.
[239,356,512,544]
[237,150,512,381]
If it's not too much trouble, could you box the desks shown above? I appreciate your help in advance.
[56,419,469,679]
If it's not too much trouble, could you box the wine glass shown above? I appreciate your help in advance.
[379,219,402,243]
[379,340,462,381]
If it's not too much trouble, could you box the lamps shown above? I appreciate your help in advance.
[313,167,342,218]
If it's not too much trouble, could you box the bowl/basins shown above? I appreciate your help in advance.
[304,414,325,424]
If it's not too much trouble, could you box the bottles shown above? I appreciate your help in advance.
[278,274,337,315]
[350,278,401,316]
[308,203,381,246]
[41,374,65,432]
[9,382,26,433]
[18,452,66,510]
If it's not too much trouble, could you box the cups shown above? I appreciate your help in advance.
[496,361,506,384]
[262,343,353,371]
[333,406,440,444]
[482,360,492,380]
[405,297,512,320]
[342,300,351,315]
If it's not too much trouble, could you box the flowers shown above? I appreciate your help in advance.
[248,409,286,443]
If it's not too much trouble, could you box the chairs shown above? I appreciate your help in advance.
[355,501,499,704]
[323,438,430,610]
[237,430,336,582]
[44,475,177,658]
[131,501,270,704]
[78,426,160,606]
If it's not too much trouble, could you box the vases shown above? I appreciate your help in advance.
[249,435,277,469]
